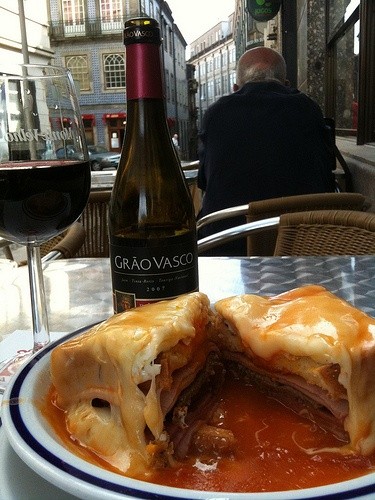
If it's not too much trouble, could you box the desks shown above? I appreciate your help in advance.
[0,256,375,500]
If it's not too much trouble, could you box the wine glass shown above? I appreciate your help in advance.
[0,64,93,394]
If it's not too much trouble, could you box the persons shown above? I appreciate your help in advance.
[196,45,334,256]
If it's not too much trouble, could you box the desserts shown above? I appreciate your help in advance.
[49,284,375,481]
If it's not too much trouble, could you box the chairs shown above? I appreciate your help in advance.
[199,210,375,257]
[0,221,87,267]
[195,191,372,257]
[15,190,112,266]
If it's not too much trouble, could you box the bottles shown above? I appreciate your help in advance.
[104,15,199,313]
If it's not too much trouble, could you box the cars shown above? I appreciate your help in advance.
[55,145,122,171]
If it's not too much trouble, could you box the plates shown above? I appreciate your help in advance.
[0,320,375,500]
